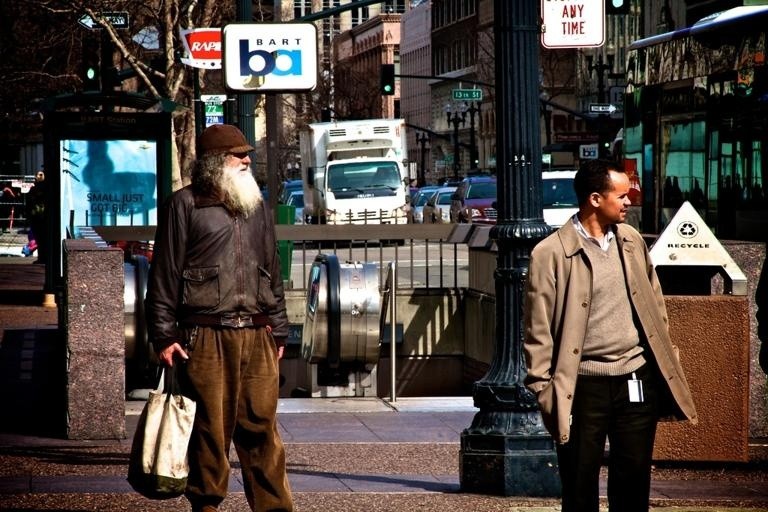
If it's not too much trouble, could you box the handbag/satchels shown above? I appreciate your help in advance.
[125,391,199,501]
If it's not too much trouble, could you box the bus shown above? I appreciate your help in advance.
[619,3,768,243]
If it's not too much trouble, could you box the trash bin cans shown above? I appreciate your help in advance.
[649,201,750,463]
[292,254,381,398]
[276,205,296,282]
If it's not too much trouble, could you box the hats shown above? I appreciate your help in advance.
[201,124,256,155]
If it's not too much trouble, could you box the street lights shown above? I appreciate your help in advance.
[445,103,466,177]
[287,162,299,182]
[415,124,429,187]
[539,93,555,147]
[585,44,616,140]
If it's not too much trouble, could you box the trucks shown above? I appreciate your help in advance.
[298,117,410,249]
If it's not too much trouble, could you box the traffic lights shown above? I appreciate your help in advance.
[82,39,99,88]
[606,0,631,14]
[603,141,610,148]
[380,63,396,96]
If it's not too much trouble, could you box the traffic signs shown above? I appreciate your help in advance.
[452,89,483,101]
[589,103,616,112]
[78,10,130,31]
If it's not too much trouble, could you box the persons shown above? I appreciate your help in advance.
[144,124,297,512]
[521,156,701,512]
[754,255,768,374]
[0,171,46,244]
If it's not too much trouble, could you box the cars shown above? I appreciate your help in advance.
[409,169,584,234]
[262,180,304,227]
